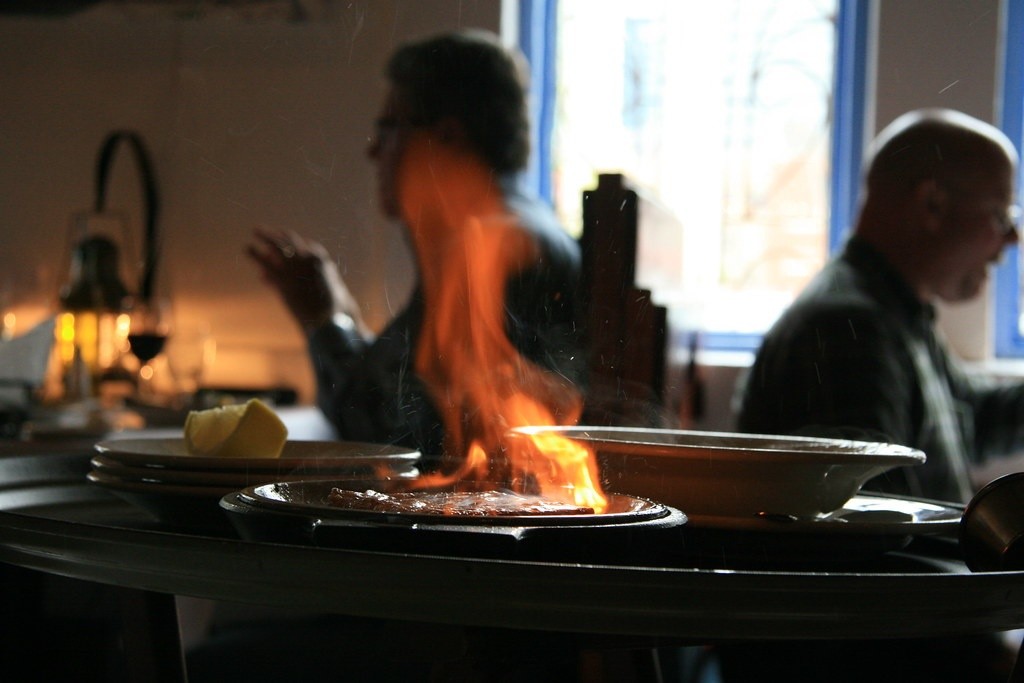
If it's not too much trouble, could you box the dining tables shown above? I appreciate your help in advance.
[0,450,1024,683]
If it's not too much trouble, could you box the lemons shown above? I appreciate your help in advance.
[185,398,287,458]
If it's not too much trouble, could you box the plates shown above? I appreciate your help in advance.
[685,490,968,544]
[220,476,686,563]
[86,438,421,504]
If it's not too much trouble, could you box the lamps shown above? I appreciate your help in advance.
[54,125,167,384]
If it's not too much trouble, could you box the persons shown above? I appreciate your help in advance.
[239,27,592,464]
[710,107,1024,683]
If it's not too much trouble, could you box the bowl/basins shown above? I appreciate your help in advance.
[504,425,923,522]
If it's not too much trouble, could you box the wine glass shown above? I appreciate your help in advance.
[125,297,169,402]
